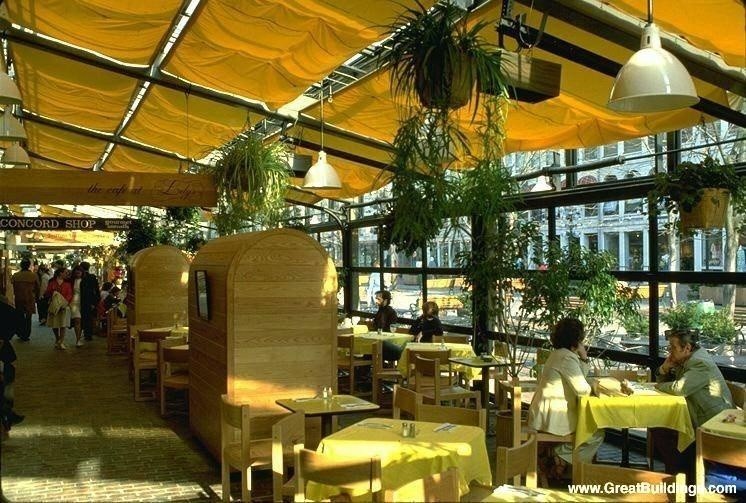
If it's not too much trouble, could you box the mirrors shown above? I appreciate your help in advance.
[194,269,210,321]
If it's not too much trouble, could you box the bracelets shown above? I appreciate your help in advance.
[581,359,589,364]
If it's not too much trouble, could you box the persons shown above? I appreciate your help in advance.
[357,290,399,392]
[13,258,128,350]
[367,261,391,308]
[382,302,444,361]
[654,329,737,474]
[527,317,606,488]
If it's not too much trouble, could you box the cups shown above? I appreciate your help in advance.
[636,368,648,385]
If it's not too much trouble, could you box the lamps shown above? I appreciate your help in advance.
[0,57,22,103]
[0,141,31,164]
[608,0,699,113]
[0,103,27,141]
[302,91,342,189]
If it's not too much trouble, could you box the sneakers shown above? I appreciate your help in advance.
[20,318,92,349]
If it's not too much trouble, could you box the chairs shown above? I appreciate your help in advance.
[105,306,189,420]
[219,326,746,503]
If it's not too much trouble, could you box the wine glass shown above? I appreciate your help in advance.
[390,324,396,336]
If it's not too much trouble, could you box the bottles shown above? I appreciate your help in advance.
[441,339,445,349]
[400,422,416,439]
[323,387,332,400]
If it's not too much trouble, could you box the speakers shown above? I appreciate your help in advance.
[476,50,561,104]
[279,155,312,178]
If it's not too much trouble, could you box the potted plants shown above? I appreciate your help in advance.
[356,0,517,255]
[636,157,746,239]
[207,116,294,220]
